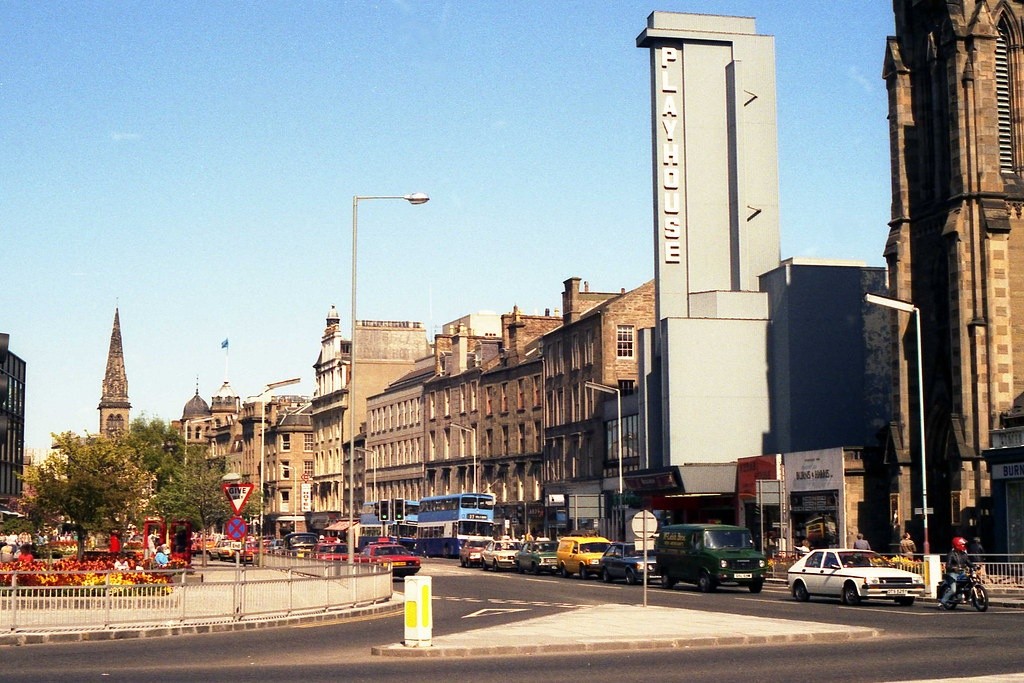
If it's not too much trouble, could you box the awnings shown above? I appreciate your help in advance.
[324,521,359,540]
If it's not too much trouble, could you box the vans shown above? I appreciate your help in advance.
[556,537,612,580]
[655,524,768,594]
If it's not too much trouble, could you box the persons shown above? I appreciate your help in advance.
[521,534,534,545]
[900,532,916,562]
[797,540,810,560]
[0,530,100,552]
[162,544,174,561]
[155,546,169,566]
[853,534,871,551]
[937,537,985,611]
[970,536,985,562]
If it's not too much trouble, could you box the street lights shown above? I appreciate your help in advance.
[181,417,213,586]
[865,294,929,554]
[585,382,623,544]
[350,193,429,569]
[450,422,476,493]
[278,464,297,532]
[354,447,377,502]
[258,378,302,568]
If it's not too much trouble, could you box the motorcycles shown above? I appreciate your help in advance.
[940,559,988,612]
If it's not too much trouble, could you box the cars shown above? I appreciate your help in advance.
[353,545,421,578]
[599,542,657,585]
[190,532,362,561]
[480,541,522,572]
[788,548,925,607]
[514,541,558,575]
[459,540,491,569]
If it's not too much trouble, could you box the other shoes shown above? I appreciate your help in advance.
[938,604,946,611]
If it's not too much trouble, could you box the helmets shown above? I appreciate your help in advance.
[952,537,968,552]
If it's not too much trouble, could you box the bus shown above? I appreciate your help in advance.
[417,493,495,559]
[359,500,420,556]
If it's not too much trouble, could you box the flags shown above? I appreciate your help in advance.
[221,339,229,349]
